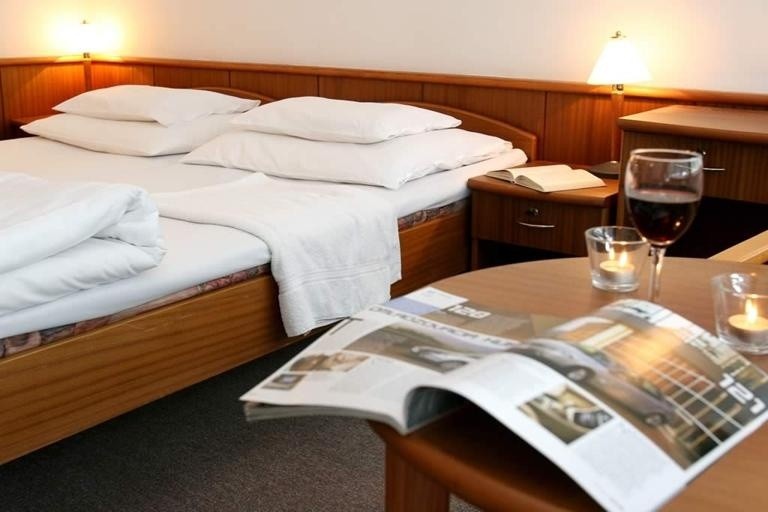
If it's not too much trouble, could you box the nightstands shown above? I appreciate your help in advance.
[467,159,621,270]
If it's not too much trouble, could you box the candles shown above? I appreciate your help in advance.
[727,312,766,346]
[597,261,636,283]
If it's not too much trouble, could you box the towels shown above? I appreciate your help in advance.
[158,169,404,338]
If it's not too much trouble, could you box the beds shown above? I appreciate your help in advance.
[1,85,538,469]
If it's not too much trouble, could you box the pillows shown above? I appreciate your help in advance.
[52,84,260,130]
[20,113,240,156]
[230,96,462,143]
[180,129,512,190]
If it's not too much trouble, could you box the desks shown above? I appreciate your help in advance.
[614,104,768,246]
[360,253,766,511]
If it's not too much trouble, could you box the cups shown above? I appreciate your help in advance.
[711,269,768,512]
[585,223,650,293]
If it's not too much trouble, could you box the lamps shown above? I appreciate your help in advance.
[584,29,651,178]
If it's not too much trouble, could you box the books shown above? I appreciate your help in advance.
[486,164,608,192]
[241,286,768,512]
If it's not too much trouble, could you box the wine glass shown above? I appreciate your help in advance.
[624,149,704,304]
